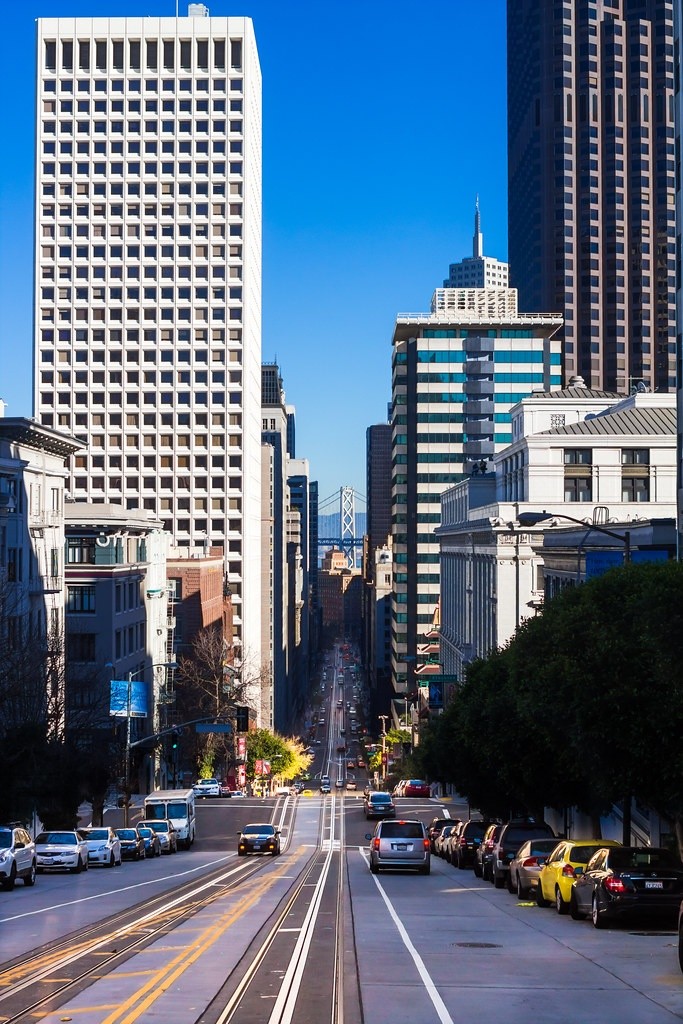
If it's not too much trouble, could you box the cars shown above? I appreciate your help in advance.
[427,816,501,869]
[113,828,145,861]
[362,786,395,818]
[192,778,244,798]
[136,827,162,858]
[570,847,683,969]
[236,824,282,856]
[392,780,430,798]
[278,782,304,795]
[474,819,625,914]
[365,818,430,875]
[33,831,89,874]
[309,639,365,793]
[75,827,121,867]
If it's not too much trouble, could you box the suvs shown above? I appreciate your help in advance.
[136,818,176,855]
[0,820,38,891]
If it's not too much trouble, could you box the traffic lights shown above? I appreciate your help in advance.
[166,732,178,750]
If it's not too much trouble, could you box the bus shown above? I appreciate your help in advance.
[144,789,195,850]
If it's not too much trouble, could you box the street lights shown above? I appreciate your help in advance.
[516,512,632,852]
[124,663,179,827]
